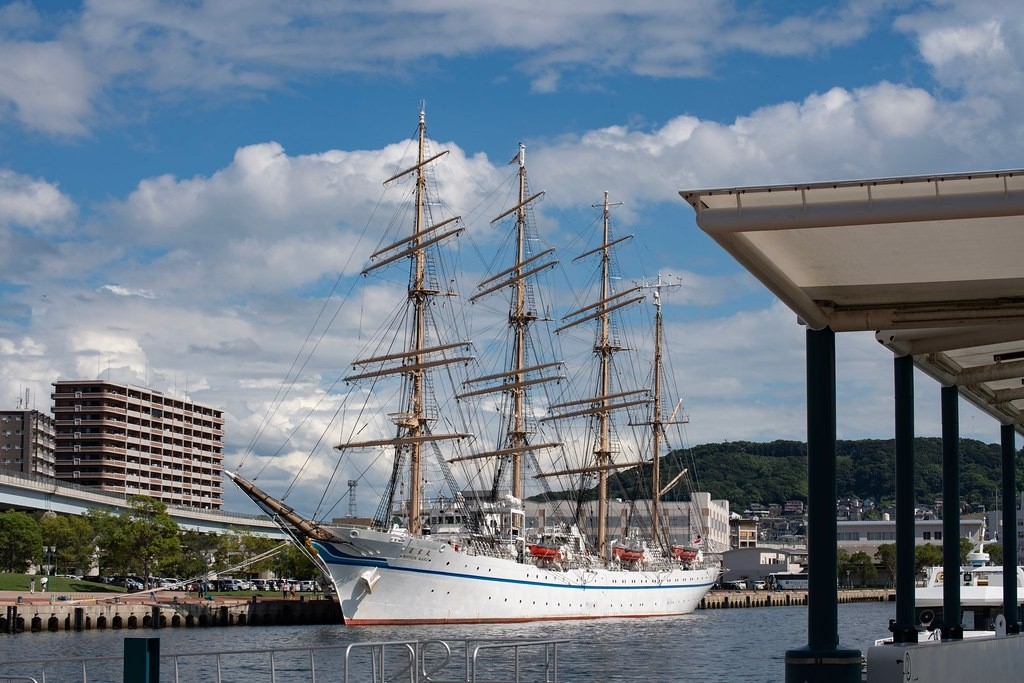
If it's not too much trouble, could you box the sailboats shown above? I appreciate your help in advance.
[221,96,734,625]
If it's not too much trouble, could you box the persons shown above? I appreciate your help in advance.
[30,576,36,594]
[150,571,333,604]
[40,576,48,593]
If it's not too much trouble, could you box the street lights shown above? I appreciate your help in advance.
[41,545,57,592]
[845,571,850,590]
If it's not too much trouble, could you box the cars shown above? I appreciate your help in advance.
[57,573,326,593]
[753,580,766,590]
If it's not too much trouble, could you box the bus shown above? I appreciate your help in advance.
[768,572,808,590]
[723,579,747,590]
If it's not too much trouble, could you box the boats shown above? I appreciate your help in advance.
[915,516,1024,633]
